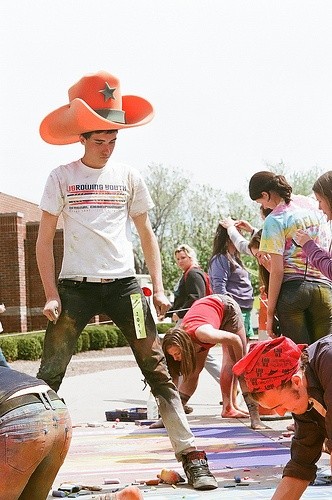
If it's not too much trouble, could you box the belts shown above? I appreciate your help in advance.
[66,276,116,283]
[0,389,62,418]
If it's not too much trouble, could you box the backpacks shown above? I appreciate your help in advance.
[185,266,213,297]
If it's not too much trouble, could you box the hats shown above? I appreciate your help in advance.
[40,69,155,144]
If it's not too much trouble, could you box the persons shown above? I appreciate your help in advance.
[0,365,72,500]
[247,229,270,298]
[0,304,10,368]
[249,171,332,434]
[233,335,332,499]
[35,118,219,490]
[295,171,332,281]
[208,217,255,419]
[149,293,272,430]
[218,204,274,257]
[158,244,240,414]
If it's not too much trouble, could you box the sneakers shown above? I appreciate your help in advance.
[181,450,218,489]
